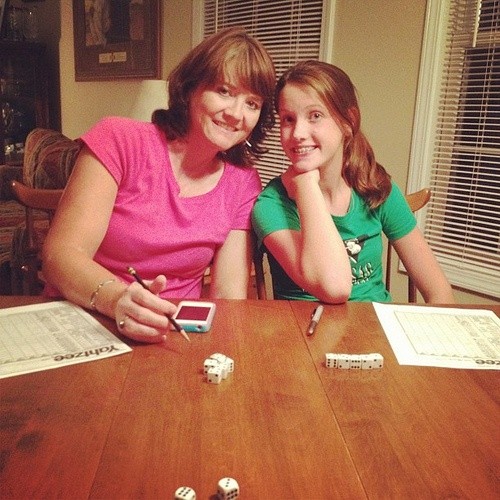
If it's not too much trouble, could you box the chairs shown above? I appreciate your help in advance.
[252,188,430,304]
[11,181,64,295]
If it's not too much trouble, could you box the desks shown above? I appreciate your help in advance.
[1,295,500,500]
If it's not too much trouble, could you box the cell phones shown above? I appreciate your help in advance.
[170,301,216,333]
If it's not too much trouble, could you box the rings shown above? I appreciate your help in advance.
[119,316,130,329]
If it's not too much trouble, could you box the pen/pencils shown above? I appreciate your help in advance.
[305,304,324,336]
[126,264,191,344]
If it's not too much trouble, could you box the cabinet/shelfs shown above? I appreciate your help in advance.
[1,38,48,167]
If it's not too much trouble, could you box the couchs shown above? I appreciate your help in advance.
[0,128,81,295]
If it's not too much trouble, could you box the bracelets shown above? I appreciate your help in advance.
[89,278,127,312]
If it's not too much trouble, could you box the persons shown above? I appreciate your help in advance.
[250,60,454,306]
[40,26,277,342]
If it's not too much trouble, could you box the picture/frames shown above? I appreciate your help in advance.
[72,0,163,82]
